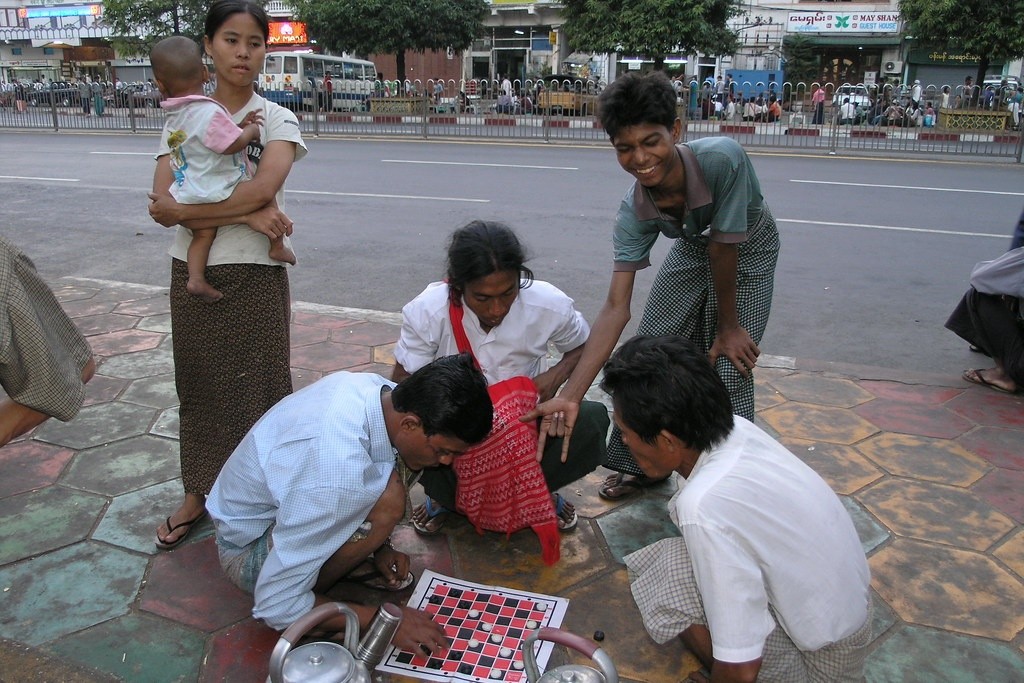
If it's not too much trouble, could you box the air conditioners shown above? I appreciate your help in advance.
[882,60,902,74]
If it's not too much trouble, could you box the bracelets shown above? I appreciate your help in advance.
[383,540,396,550]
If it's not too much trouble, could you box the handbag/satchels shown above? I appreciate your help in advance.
[448,280,562,567]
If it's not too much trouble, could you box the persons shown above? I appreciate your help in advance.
[93,76,105,116]
[149,37,297,303]
[563,69,629,95]
[374,72,385,98]
[146,0,309,547]
[79,74,158,92]
[0,234,95,447]
[78,77,93,115]
[517,69,781,503]
[944,209,1024,394]
[812,82,825,125]
[497,71,542,115]
[602,334,873,683]
[320,71,333,115]
[433,77,443,101]
[670,73,795,123]
[205,352,493,659]
[0,74,52,114]
[828,76,1024,130]
[389,219,610,538]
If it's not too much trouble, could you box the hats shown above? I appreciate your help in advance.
[915,80,919,84]
[893,100,900,104]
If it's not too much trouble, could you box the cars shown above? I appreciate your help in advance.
[25,80,84,108]
[980,79,1019,107]
[829,84,879,125]
[535,73,604,117]
[132,85,164,109]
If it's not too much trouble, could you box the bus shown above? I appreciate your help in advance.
[257,51,383,114]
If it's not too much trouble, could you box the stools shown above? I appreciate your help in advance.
[464,106,478,115]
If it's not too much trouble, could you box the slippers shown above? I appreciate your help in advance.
[549,492,577,531]
[154,509,209,549]
[341,556,415,591]
[599,469,671,500]
[303,628,349,641]
[413,496,453,534]
[962,369,1015,393]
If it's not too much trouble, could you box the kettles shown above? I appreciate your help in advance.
[522,627,620,683]
[265,601,404,683]
